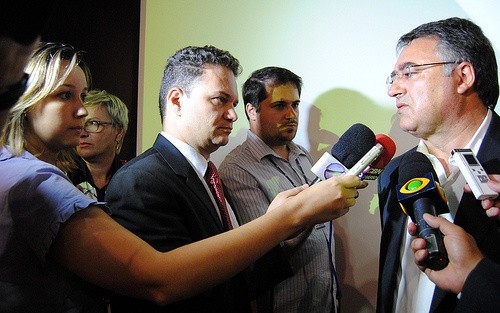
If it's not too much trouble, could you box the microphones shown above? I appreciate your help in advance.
[310,124,499,271]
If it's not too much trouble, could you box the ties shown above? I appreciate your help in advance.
[205,161,233,230]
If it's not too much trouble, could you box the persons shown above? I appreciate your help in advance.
[375,17,500,313]
[463,174,500,217]
[102,45,310,313]
[408,213,500,313]
[0,41,360,313]
[217,67,369,313]
[60,90,128,209]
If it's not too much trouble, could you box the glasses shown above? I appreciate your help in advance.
[385,62,461,87]
[84,121,122,132]
